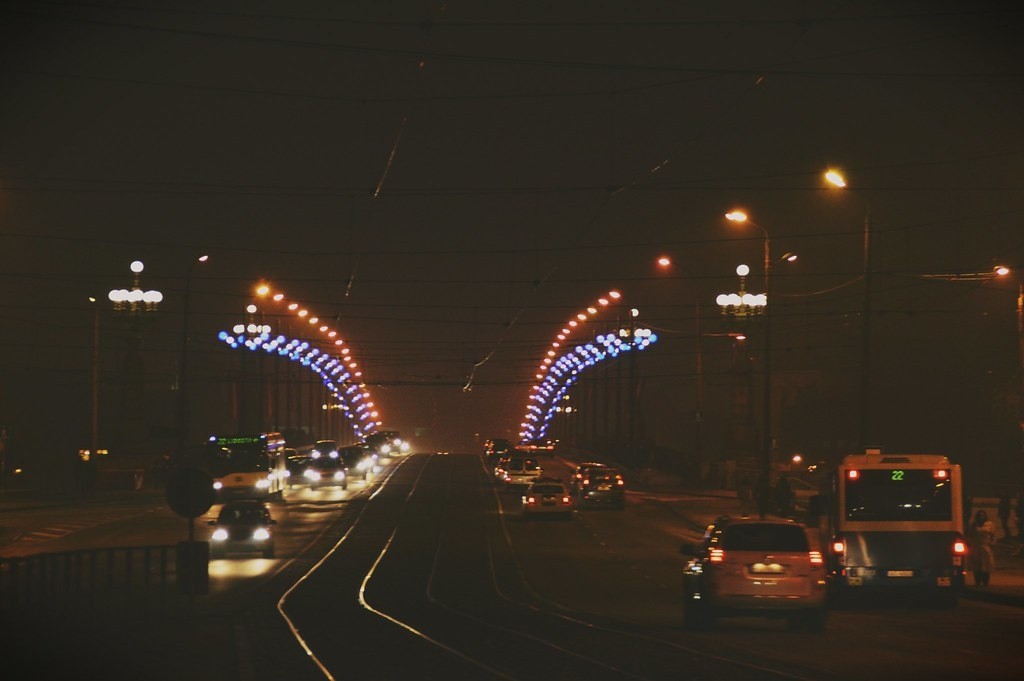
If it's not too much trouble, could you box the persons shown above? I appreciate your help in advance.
[968,510,995,593]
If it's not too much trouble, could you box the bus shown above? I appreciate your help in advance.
[817,452,971,612]
[200,432,286,507]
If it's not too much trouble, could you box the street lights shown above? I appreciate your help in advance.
[177,251,210,485]
[108,260,163,465]
[521,287,640,471]
[656,258,749,489]
[245,279,380,446]
[821,166,872,449]
[995,265,1024,434]
[724,209,798,513]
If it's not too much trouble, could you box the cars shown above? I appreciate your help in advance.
[208,501,279,560]
[781,476,820,512]
[339,429,400,479]
[483,435,625,516]
[309,438,339,460]
[307,458,349,489]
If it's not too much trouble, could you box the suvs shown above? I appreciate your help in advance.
[677,513,827,631]
